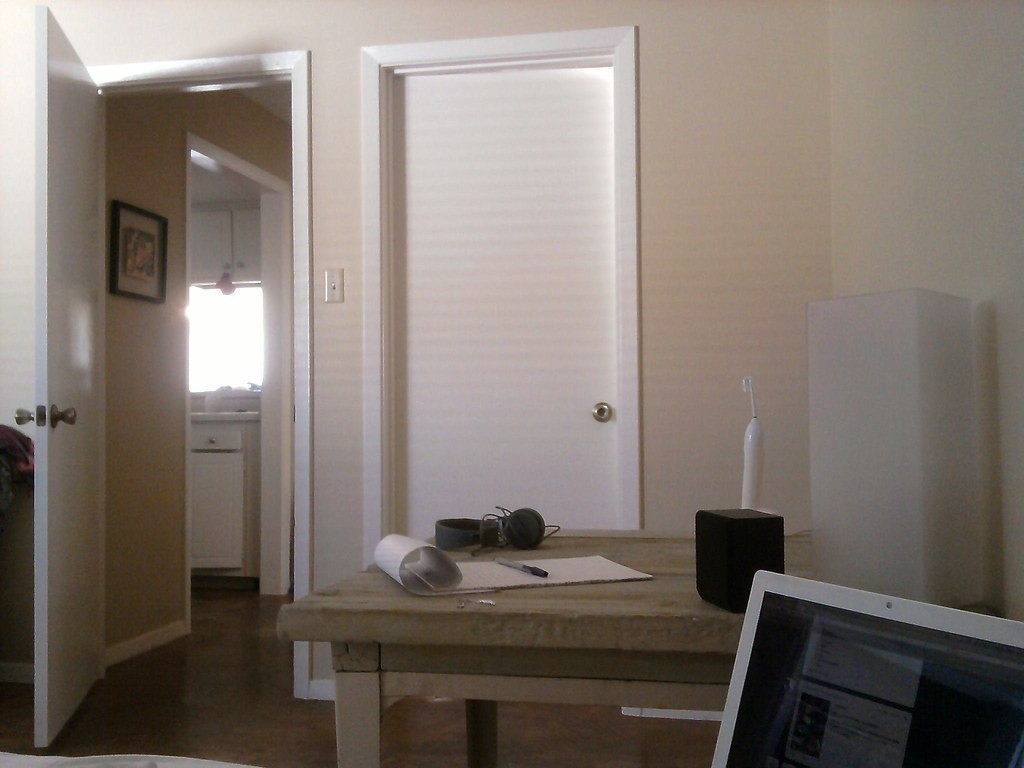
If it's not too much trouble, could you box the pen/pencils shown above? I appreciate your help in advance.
[491,552,552,580]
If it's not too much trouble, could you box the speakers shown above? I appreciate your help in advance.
[695,509,784,614]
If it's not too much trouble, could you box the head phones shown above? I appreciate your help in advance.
[434,509,546,549]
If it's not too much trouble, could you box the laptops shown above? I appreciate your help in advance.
[711,570,1024,768]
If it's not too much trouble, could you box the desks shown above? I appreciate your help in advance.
[277,529,997,767]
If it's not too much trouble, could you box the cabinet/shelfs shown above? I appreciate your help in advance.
[191,202,262,288]
[190,412,261,581]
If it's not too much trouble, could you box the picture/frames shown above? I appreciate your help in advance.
[108,201,168,303]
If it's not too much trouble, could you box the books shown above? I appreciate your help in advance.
[374,533,654,596]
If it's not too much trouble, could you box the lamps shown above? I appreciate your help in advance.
[811,293,983,608]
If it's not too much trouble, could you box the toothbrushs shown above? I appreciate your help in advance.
[741,377,763,511]
